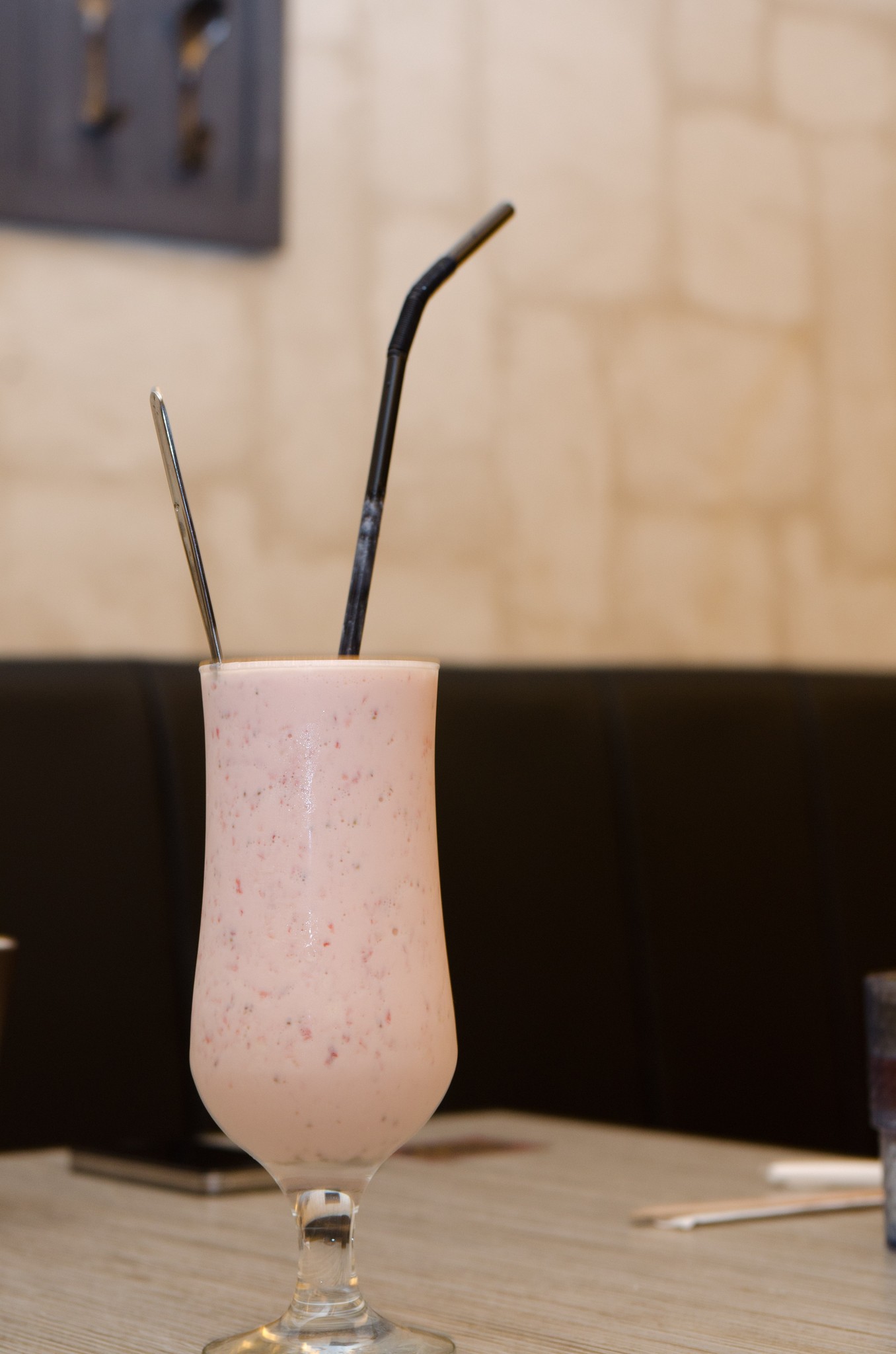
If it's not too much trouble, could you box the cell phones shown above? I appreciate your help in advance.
[71,1139,280,1194]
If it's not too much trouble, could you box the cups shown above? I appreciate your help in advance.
[864,971,896,1251]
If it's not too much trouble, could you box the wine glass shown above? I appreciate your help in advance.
[182,658,469,1353]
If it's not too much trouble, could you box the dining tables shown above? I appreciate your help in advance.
[4,1100,896,1354]
